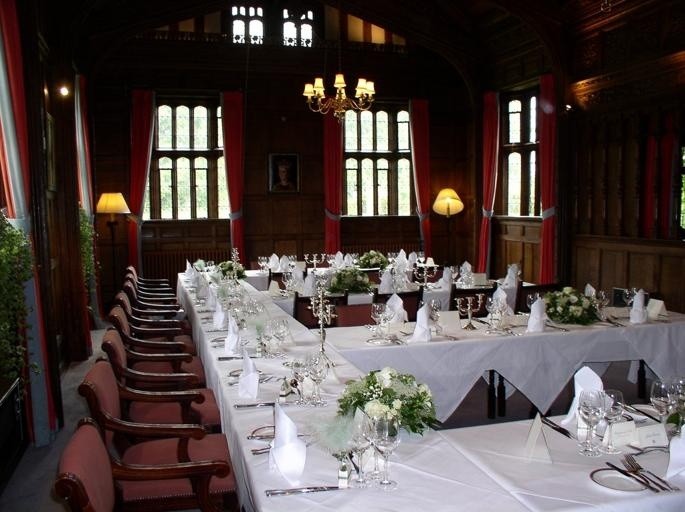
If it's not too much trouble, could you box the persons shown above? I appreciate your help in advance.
[272,159,295,191]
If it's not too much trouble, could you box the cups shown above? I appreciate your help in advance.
[526,295,538,309]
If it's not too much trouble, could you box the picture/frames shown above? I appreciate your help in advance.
[267,149,302,196]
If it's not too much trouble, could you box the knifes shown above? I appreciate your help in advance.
[233,402,276,409]
[626,402,661,424]
[542,416,576,440]
[605,460,658,493]
[264,486,337,496]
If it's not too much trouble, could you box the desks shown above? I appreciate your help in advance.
[173,255,685,507]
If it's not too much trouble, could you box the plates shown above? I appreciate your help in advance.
[631,403,661,420]
[590,468,650,491]
[366,337,390,347]
[251,426,276,440]
[229,367,262,377]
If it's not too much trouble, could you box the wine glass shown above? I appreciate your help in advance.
[448,264,473,289]
[197,258,263,325]
[262,316,289,359]
[257,254,297,274]
[325,253,357,267]
[576,390,623,458]
[652,376,685,441]
[386,251,427,266]
[419,297,441,337]
[371,303,396,342]
[486,296,508,336]
[589,288,647,327]
[341,410,400,492]
[290,353,329,408]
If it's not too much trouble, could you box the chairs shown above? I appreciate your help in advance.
[51,256,237,505]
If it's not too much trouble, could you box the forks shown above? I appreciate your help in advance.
[622,453,679,494]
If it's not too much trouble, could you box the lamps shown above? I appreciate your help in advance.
[95,191,133,269]
[303,71,378,124]
[432,186,466,222]
